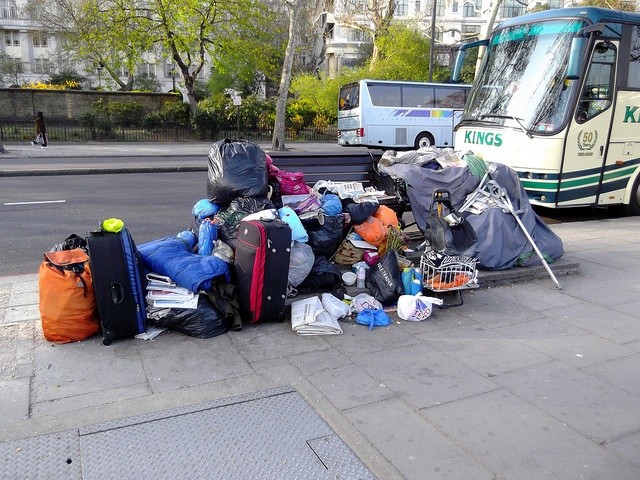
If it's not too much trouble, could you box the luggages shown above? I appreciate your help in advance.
[85,220,147,345]
[233,217,292,325]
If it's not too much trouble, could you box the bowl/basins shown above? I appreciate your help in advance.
[342,271,357,286]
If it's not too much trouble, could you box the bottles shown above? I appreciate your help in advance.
[357,261,367,290]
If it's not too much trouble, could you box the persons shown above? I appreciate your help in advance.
[30,111,50,149]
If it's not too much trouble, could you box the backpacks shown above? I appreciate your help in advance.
[39,248,101,345]
[296,271,347,300]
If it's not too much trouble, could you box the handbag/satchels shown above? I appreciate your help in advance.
[45,133,48,144]
[270,164,308,195]
[427,198,478,256]
[35,133,44,144]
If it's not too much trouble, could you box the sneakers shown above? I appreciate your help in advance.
[31,142,34,147]
[41,146,47,149]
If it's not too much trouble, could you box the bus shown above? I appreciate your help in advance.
[338,79,503,150]
[452,6,639,215]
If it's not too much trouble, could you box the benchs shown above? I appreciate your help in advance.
[262,150,413,231]
